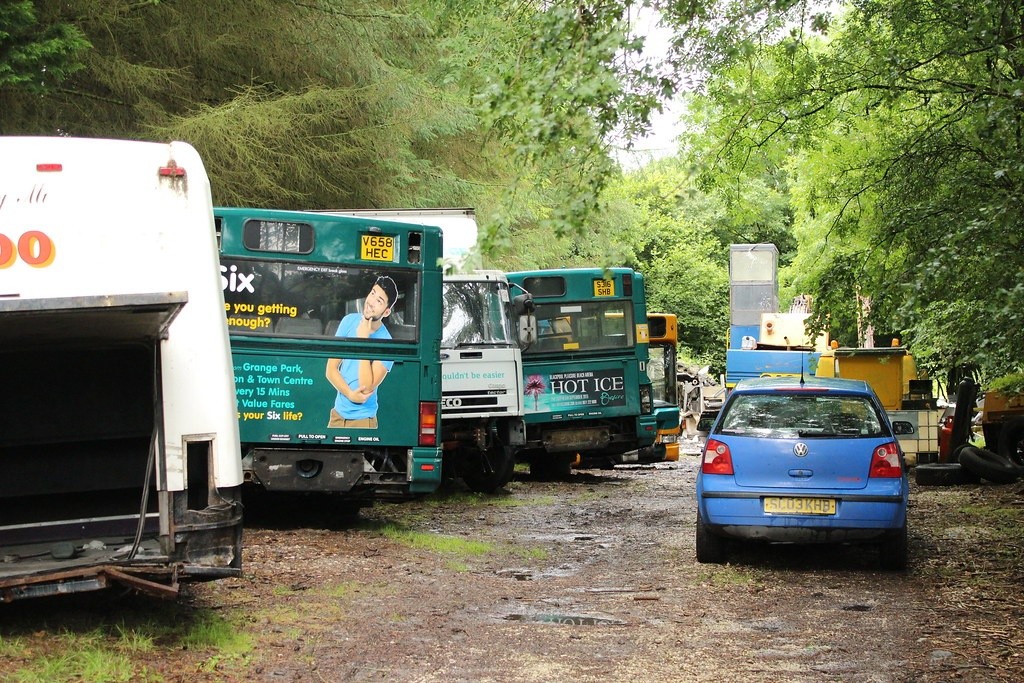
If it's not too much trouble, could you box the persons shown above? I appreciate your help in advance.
[325,275,399,429]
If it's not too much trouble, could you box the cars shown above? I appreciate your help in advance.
[692,375,911,575]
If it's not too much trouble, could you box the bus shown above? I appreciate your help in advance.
[552,313,683,462]
[500,268,669,477]
[210,207,443,520]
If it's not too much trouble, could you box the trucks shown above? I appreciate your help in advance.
[306,208,539,497]
[0,134,243,604]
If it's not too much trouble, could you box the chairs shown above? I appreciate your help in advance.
[747,401,795,428]
[828,411,862,434]
[591,334,627,346]
[385,323,416,339]
[539,337,568,350]
[274,317,323,336]
[324,320,341,336]
[228,315,273,333]
